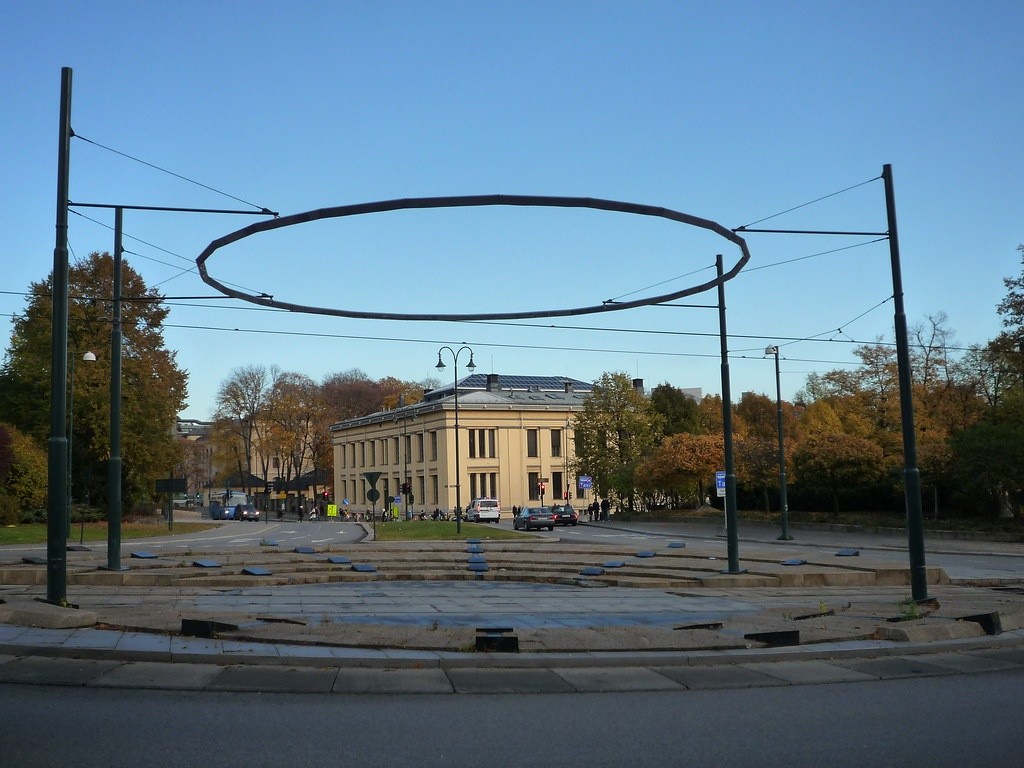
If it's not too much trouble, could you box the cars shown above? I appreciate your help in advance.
[452,514,466,522]
[173,493,204,508]
[551,504,578,526]
[513,506,556,532]
[232,503,260,523]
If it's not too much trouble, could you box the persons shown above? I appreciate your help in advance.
[309,507,321,522]
[513,505,525,518]
[297,505,304,522]
[365,507,371,522]
[354,513,360,522]
[553,502,571,508]
[380,508,393,522]
[185,498,203,511]
[588,498,620,522]
[339,507,349,522]
[406,506,463,521]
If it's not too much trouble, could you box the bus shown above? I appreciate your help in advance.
[209,491,249,521]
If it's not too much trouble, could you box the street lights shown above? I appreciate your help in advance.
[564,416,571,505]
[315,431,334,521]
[393,405,417,520]
[764,344,795,540]
[65,350,98,540]
[434,346,477,534]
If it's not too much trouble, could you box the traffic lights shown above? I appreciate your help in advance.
[399,483,405,494]
[541,484,545,495]
[227,489,234,499]
[536,485,540,495]
[265,485,271,495]
[564,492,568,500]
[569,492,572,500]
[405,484,412,494]
[323,491,328,501]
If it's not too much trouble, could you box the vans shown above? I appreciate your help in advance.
[466,496,501,523]
